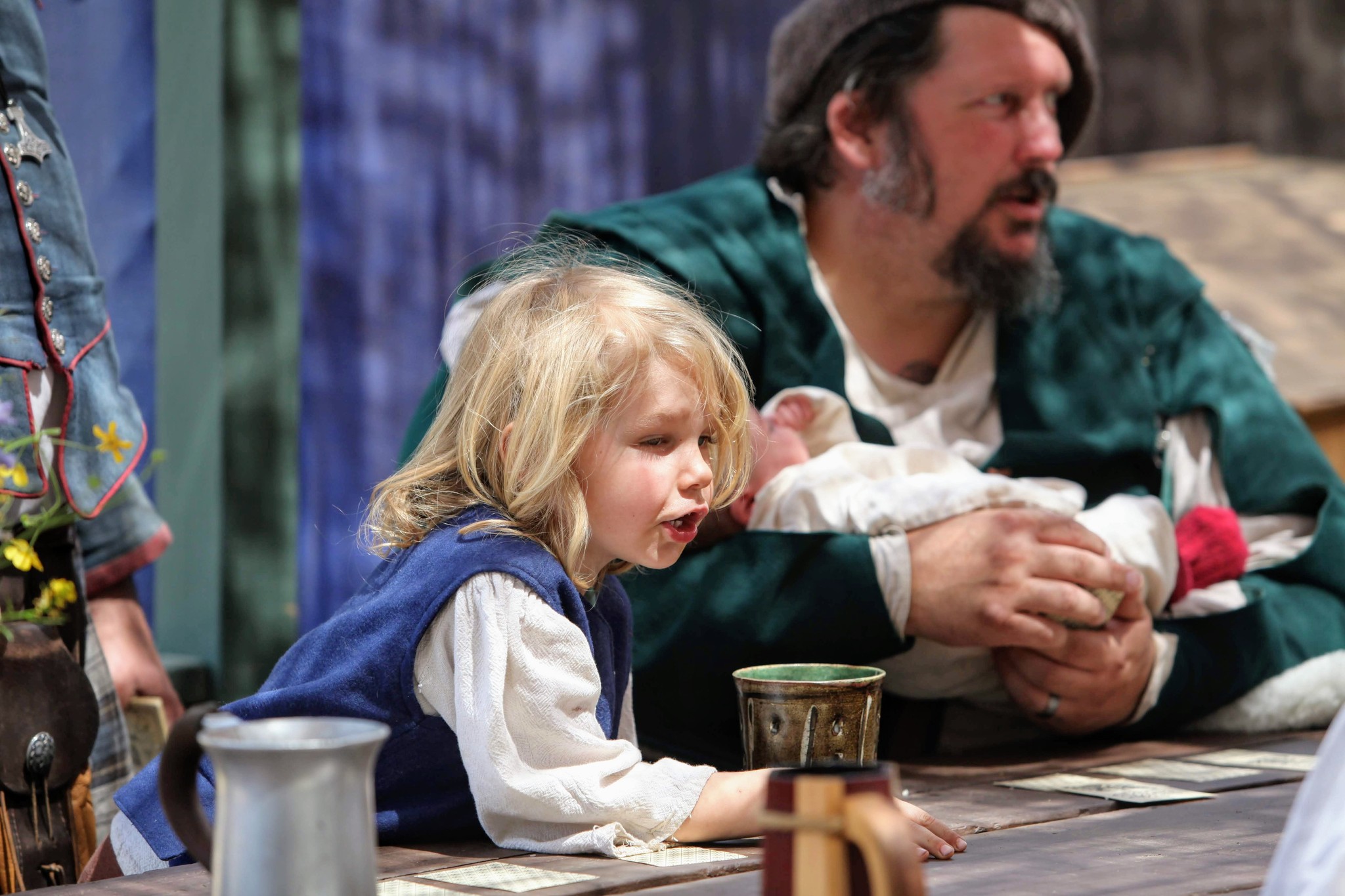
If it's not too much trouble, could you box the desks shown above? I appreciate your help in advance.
[2,715,1332,895]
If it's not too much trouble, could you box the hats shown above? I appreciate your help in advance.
[763,0,1101,165]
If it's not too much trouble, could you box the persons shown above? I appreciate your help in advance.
[77,223,970,885]
[0,0,183,894]
[396,0,1345,770]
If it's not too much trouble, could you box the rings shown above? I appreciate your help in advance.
[1038,696,1058,718]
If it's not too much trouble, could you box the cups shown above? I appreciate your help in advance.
[762,767,926,896]
[198,715,390,896]
[732,663,886,772]
